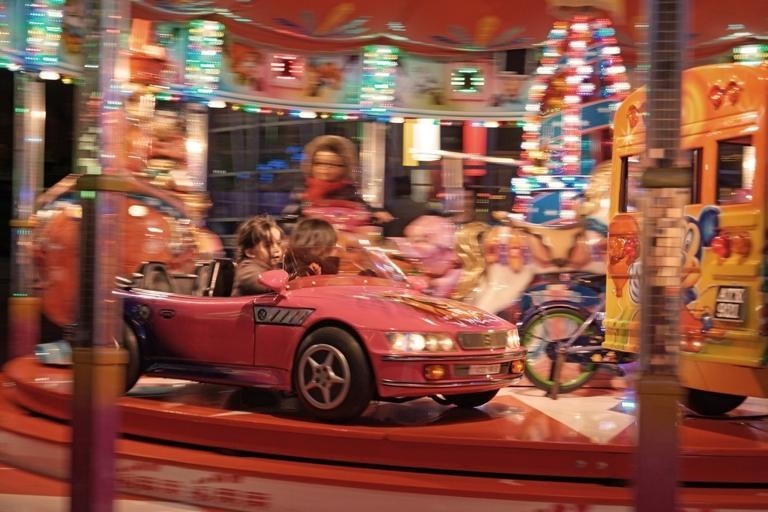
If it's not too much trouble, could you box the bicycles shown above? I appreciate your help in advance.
[520,275,609,397]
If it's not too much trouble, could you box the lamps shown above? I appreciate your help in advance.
[407,118,443,159]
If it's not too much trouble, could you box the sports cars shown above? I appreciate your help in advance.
[117,260,520,418]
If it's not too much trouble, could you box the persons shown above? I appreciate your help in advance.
[283,216,338,277]
[231,213,321,297]
[284,133,395,235]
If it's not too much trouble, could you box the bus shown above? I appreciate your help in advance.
[603,65,767,416]
